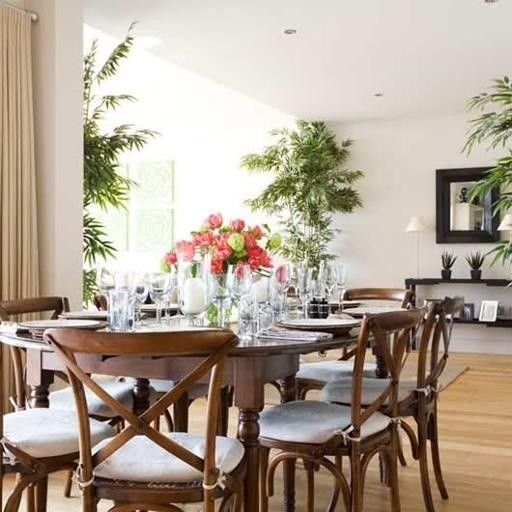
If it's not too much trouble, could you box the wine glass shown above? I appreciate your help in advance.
[106,259,348,341]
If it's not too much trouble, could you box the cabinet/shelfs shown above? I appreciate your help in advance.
[454,202,485,233]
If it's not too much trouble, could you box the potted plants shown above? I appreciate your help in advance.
[440,253,458,279]
[465,251,485,280]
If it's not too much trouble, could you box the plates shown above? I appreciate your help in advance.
[341,306,408,317]
[279,317,360,328]
[18,320,100,331]
[59,311,107,318]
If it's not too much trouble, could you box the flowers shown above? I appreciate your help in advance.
[158,212,281,322]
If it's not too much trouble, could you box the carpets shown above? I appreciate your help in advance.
[264,348,469,407]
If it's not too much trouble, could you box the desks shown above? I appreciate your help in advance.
[403,279,512,352]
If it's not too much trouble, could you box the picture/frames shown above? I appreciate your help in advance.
[478,300,499,322]
[433,166,501,243]
[460,304,474,321]
